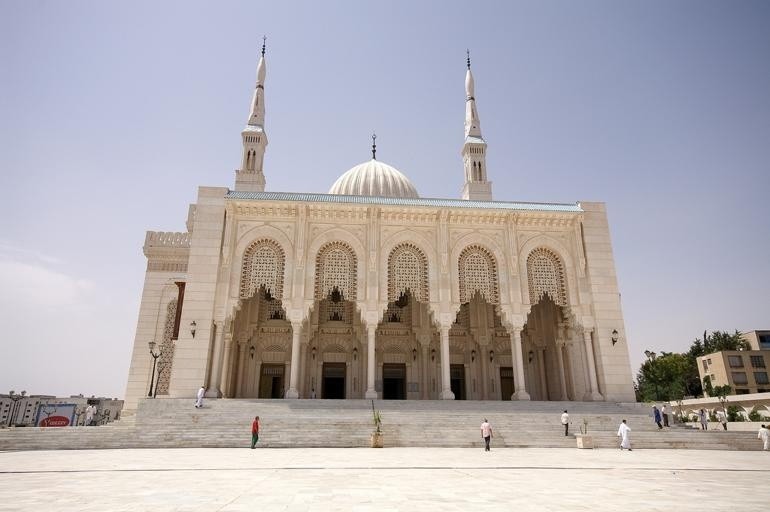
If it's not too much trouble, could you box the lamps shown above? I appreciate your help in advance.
[528,349,534,363]
[190,319,197,337]
[352,346,359,360]
[470,349,495,363]
[249,344,256,360]
[411,346,436,363]
[312,346,317,360]
[612,329,619,347]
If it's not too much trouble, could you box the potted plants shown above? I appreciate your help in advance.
[368,398,387,448]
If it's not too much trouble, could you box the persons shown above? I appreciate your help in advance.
[250,416,259,448]
[758,424,770,451]
[194,386,205,408]
[617,420,633,451]
[661,404,671,427]
[713,410,728,431]
[694,409,708,431]
[652,405,663,430]
[310,388,317,399]
[84,402,97,425]
[481,419,494,451]
[561,409,573,436]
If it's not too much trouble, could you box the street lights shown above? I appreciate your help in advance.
[147,339,164,399]
[6,390,27,428]
